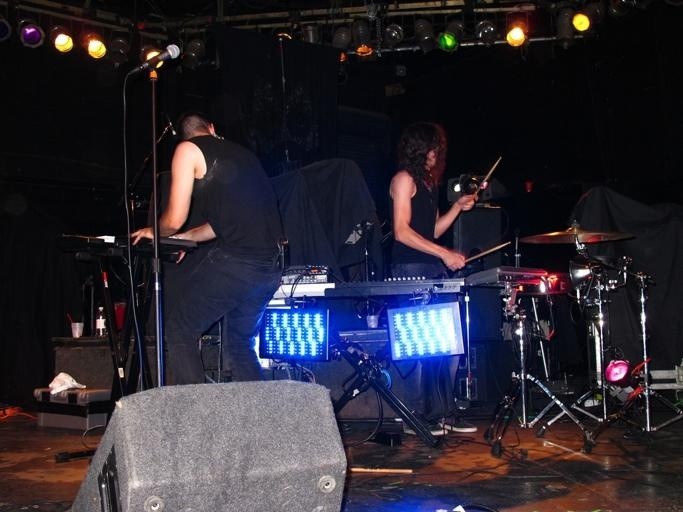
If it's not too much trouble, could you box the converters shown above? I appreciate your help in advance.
[376,431,400,445]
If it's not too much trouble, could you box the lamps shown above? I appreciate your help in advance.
[0,0,174,76]
[172,1,630,76]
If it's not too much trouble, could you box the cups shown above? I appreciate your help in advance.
[71,322,84,339]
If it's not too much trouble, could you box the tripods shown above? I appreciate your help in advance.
[54,58,167,463]
[484,236,682,459]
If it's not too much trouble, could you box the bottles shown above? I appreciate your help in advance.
[96,307,108,338]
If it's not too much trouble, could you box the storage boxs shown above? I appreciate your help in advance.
[31,385,110,435]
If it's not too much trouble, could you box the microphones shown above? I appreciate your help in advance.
[141,44,180,69]
[164,112,176,136]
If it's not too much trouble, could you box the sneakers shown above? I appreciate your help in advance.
[403,419,449,437]
[437,415,478,434]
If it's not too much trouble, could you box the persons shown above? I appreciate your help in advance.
[386,120,478,437]
[128,111,285,387]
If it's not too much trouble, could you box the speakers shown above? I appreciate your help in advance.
[70,381,348,512]
[53,338,157,400]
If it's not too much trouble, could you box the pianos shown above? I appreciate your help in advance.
[324,276,467,297]
[60,231,199,255]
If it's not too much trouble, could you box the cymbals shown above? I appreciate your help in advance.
[518,227,633,245]
